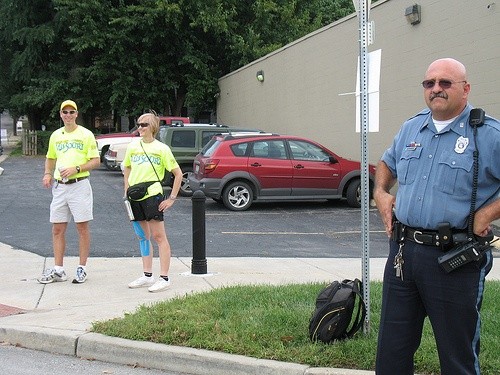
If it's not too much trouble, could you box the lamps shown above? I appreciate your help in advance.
[405,3,422,26]
[256,70,264,82]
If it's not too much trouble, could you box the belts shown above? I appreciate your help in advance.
[391,214,470,252]
[55,175,88,184]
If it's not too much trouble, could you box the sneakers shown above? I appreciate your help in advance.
[71,265,89,283]
[148,275,172,293]
[128,273,156,289]
[36,266,69,284]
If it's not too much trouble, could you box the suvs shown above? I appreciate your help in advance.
[96,122,222,163]
[158,124,269,197]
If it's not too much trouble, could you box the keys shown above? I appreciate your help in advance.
[393,243,404,280]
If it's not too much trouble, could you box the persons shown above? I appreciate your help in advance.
[37,100,100,284]
[373,58,500,375]
[123,113,183,292]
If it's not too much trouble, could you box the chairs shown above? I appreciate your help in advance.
[271,143,281,157]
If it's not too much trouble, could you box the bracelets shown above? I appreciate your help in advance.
[45,173,52,175]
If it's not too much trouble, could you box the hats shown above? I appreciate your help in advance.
[60,100,78,111]
[421,79,467,89]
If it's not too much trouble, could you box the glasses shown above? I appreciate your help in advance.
[61,110,77,114]
[137,123,150,128]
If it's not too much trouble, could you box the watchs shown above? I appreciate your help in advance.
[76,166,80,173]
[171,195,175,200]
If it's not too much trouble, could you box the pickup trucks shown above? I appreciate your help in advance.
[96,117,190,140]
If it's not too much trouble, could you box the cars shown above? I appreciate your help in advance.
[103,144,135,167]
[189,131,376,211]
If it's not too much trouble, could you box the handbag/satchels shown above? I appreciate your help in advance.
[127,181,162,200]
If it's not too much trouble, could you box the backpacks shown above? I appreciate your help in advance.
[308,276,367,345]
